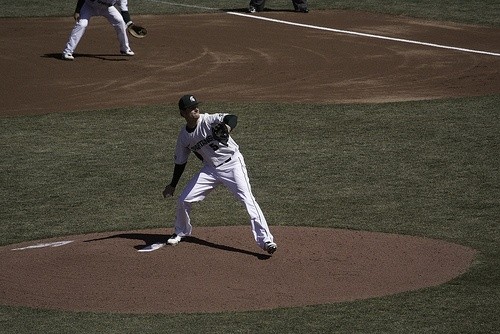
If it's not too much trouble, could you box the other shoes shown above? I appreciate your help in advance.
[120,49,134,56]
[296,8,309,13]
[62,52,74,60]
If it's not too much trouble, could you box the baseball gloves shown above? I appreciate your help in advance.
[128,23,148,39]
[210,120,230,148]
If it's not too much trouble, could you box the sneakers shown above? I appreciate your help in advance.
[167,233,183,245]
[263,241,278,254]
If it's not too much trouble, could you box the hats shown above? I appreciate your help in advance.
[179,95,201,115]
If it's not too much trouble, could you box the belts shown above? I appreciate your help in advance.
[217,157,231,167]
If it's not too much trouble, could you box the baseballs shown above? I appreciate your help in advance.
[166,194,172,198]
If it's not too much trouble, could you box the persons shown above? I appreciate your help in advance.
[248,0,309,13]
[162,95,277,254]
[62,0,147,60]
